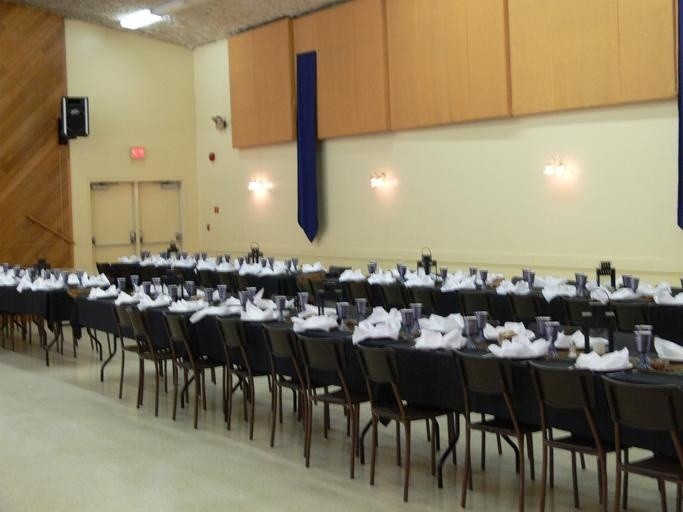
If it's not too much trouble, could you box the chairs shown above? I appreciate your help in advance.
[0,252,227,431]
[227,259,683,512]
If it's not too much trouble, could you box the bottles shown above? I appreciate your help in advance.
[566,339,577,359]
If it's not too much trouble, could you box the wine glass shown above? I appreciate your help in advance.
[620,275,639,293]
[0,247,560,360]
[631,323,655,370]
[572,272,587,298]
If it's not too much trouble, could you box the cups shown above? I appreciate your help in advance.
[592,339,605,356]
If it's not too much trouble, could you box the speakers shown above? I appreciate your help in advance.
[61,96,89,139]
